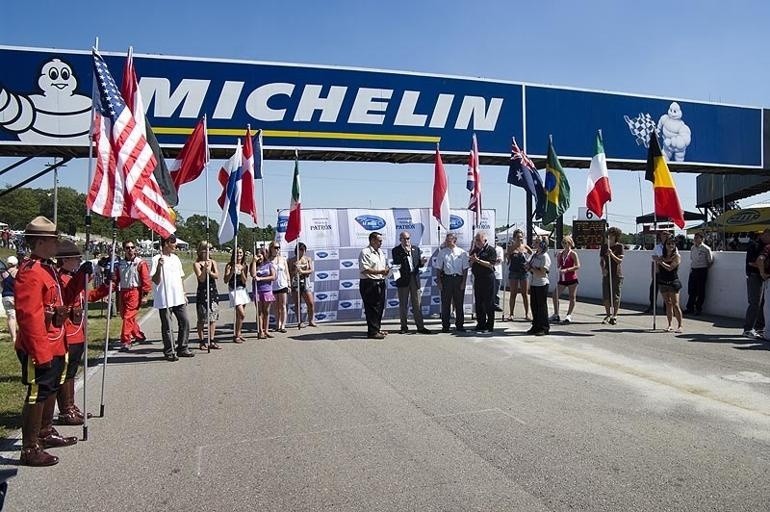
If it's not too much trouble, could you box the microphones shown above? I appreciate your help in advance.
[405,243,410,255]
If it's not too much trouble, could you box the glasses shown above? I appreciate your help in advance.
[271,246,280,249]
[666,242,675,246]
[401,238,410,240]
[124,245,136,250]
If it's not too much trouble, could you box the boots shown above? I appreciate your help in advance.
[56,375,93,426]
[39,392,79,450]
[18,400,60,467]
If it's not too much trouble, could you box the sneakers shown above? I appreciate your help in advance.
[276,328,282,332]
[475,329,494,337]
[601,316,610,325]
[309,322,317,327]
[442,324,450,332]
[258,333,266,339]
[663,325,674,332]
[281,328,288,333]
[526,325,550,336]
[207,340,222,349]
[176,350,196,358]
[682,309,694,315]
[398,329,409,334]
[742,329,760,338]
[164,353,180,362]
[376,329,389,335]
[368,332,385,339]
[232,335,247,344]
[507,316,515,321]
[300,322,306,328]
[265,332,275,338]
[131,337,147,347]
[199,341,208,350]
[563,314,572,323]
[675,328,684,333]
[118,345,132,353]
[471,325,486,332]
[548,314,561,322]
[755,330,770,339]
[694,311,702,316]
[456,325,466,332]
[610,318,617,325]
[417,328,432,334]
[525,317,533,321]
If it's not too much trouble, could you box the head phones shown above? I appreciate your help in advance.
[540,238,546,250]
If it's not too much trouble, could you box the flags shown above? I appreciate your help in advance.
[645,132,684,230]
[542,135,570,225]
[507,136,545,220]
[586,130,611,218]
[84,46,301,245]
[434,144,447,225]
[467,133,480,226]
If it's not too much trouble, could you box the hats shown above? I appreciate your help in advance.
[18,214,62,239]
[6,255,19,267]
[57,241,82,259]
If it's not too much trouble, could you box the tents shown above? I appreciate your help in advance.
[172,237,190,249]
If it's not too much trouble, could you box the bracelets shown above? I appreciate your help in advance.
[382,270,383,274]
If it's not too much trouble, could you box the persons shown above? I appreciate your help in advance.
[392,231,431,333]
[600,227,624,325]
[729,229,770,339]
[507,229,579,336]
[645,230,713,332]
[150,234,195,361]
[193,245,222,349]
[358,232,389,338]
[435,232,504,333]
[0,216,159,466]
[249,243,317,339]
